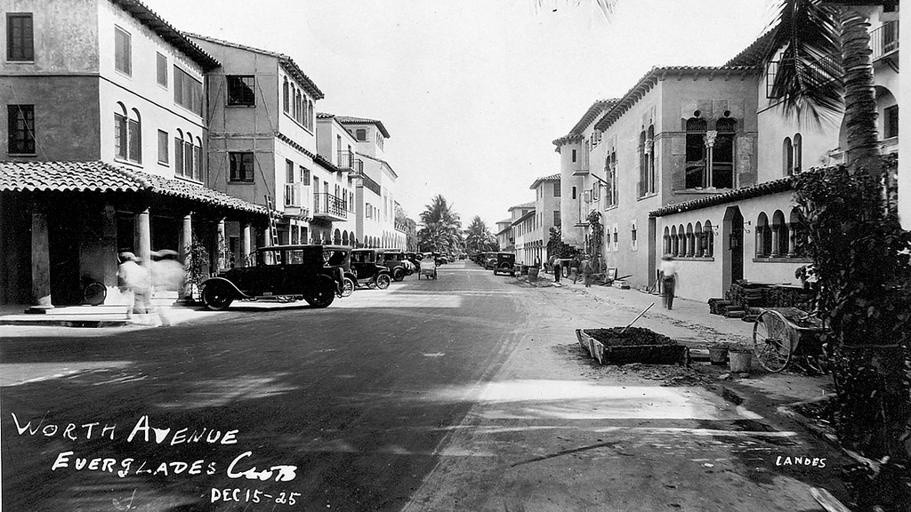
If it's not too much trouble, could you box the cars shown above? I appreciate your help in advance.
[195,238,454,311]
[458,250,517,276]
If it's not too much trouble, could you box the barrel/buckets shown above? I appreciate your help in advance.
[527,266,540,281]
[707,343,728,364]
[727,348,753,374]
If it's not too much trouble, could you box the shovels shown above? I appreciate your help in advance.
[607,302,654,344]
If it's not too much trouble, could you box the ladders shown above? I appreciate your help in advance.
[264,194,288,303]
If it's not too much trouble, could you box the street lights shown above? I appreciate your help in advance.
[642,139,655,196]
[608,162,616,207]
[703,129,717,191]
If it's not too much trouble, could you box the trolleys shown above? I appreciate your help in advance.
[750,303,832,376]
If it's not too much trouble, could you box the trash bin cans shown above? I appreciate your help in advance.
[528,266,539,282]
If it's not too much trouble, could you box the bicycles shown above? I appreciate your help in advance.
[54,260,108,306]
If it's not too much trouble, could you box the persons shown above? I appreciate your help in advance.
[552,255,562,283]
[534,256,541,265]
[580,254,594,288]
[658,252,679,310]
[116,247,149,321]
